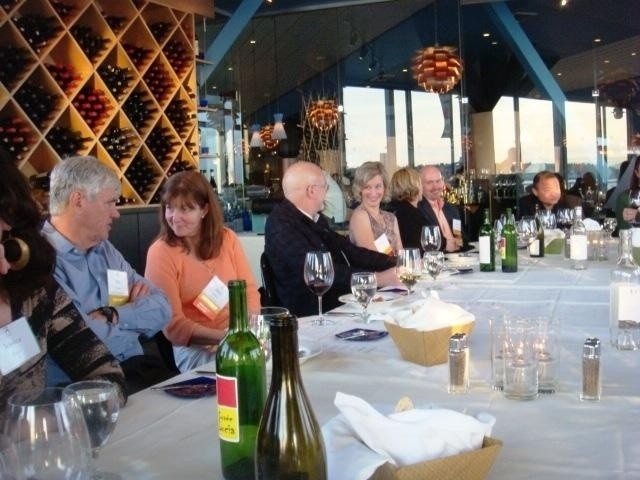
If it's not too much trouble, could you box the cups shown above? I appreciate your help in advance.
[248,306,292,394]
[565,230,608,260]
[582,336,605,404]
[627,187,640,225]
[491,309,559,400]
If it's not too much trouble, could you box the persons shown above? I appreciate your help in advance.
[517,171,573,229]
[35,153,178,397]
[0,153,130,451]
[349,162,479,255]
[319,169,347,224]
[259,161,424,319]
[606,155,640,237]
[144,168,264,375]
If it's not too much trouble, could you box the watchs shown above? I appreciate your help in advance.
[97,304,117,325]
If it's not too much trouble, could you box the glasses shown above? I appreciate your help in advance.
[306,183,329,191]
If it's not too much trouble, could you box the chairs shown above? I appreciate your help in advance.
[256,250,309,316]
[152,330,180,373]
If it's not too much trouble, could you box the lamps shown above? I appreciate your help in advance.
[262,93,282,149]
[411,1,465,92]
[310,55,338,131]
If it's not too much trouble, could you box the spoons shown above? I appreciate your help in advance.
[147,384,216,391]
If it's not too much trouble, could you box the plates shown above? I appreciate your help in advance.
[334,328,387,342]
[254,336,324,366]
[337,292,401,305]
[162,376,219,400]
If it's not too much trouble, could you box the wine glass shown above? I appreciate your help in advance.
[60,378,122,454]
[395,224,478,303]
[492,179,517,198]
[348,273,380,326]
[4,387,94,479]
[495,184,621,247]
[303,251,336,327]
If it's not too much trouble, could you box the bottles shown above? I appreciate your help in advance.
[479,207,495,272]
[199,169,217,196]
[502,206,517,273]
[609,226,640,351]
[258,314,329,479]
[216,280,266,478]
[529,203,545,259]
[448,332,473,394]
[570,205,589,271]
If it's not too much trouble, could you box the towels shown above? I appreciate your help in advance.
[391,283,476,334]
[317,381,497,480]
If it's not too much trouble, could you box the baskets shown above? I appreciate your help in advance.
[372,435,503,480]
[384,320,477,367]
